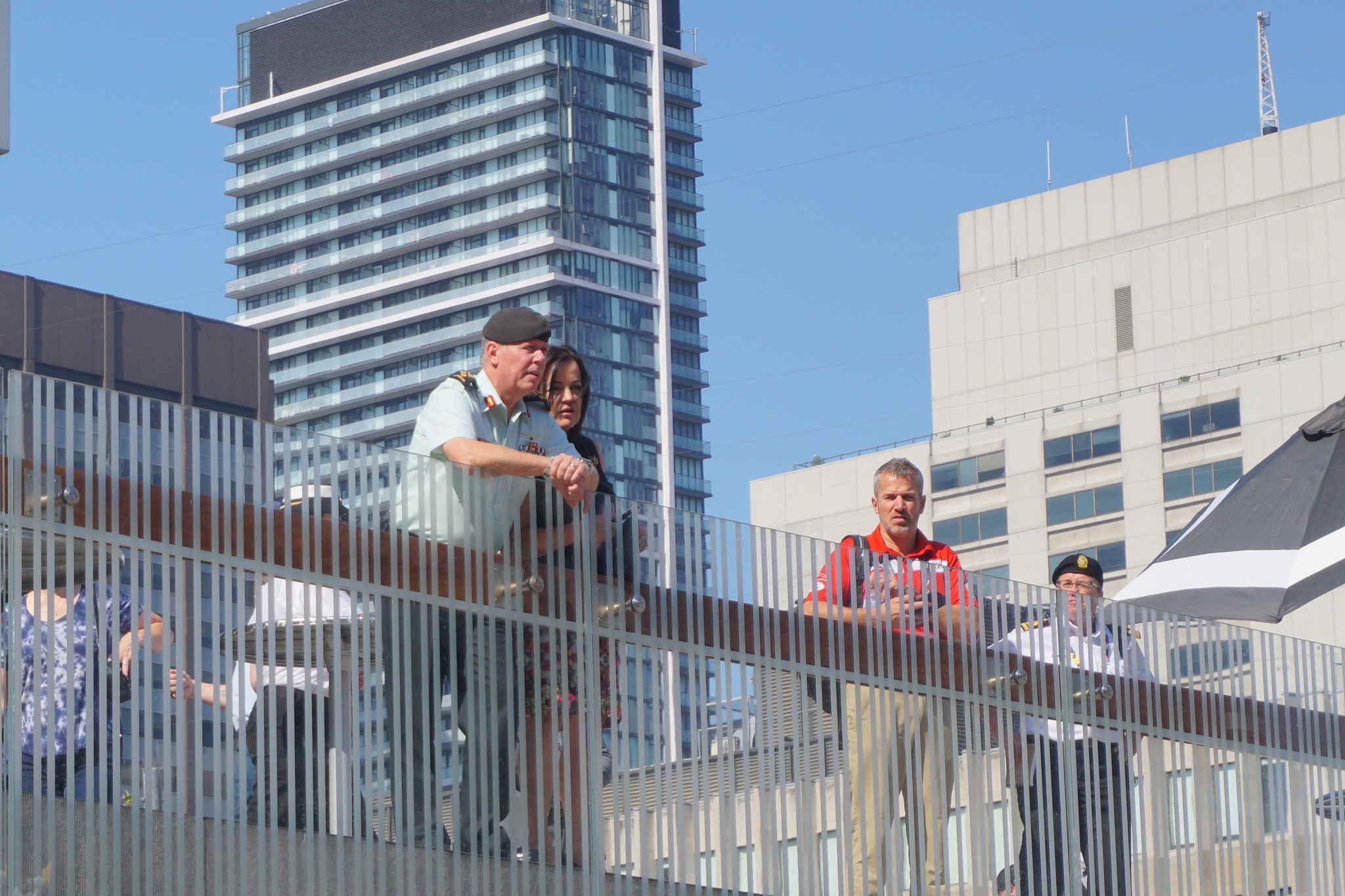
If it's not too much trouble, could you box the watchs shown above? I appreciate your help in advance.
[576,457,594,477]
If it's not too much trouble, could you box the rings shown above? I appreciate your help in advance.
[571,464,578,470]
[122,661,130,664]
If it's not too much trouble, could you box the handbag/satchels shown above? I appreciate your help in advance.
[94,652,132,704]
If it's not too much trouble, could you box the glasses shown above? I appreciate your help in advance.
[1056,580,1100,592]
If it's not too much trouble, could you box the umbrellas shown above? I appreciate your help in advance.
[1103,394,1345,629]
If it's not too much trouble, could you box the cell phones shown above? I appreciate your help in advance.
[913,591,946,614]
[613,505,640,523]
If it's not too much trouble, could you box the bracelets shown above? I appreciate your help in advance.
[545,456,557,476]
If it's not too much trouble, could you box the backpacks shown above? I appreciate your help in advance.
[801,534,876,747]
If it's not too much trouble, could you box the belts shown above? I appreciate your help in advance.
[1027,734,1105,751]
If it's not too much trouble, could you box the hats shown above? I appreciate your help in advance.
[482,306,552,345]
[1052,553,1103,587]
[281,485,349,521]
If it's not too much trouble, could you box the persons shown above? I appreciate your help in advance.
[0,307,647,870]
[804,457,981,896]
[982,553,1156,896]
